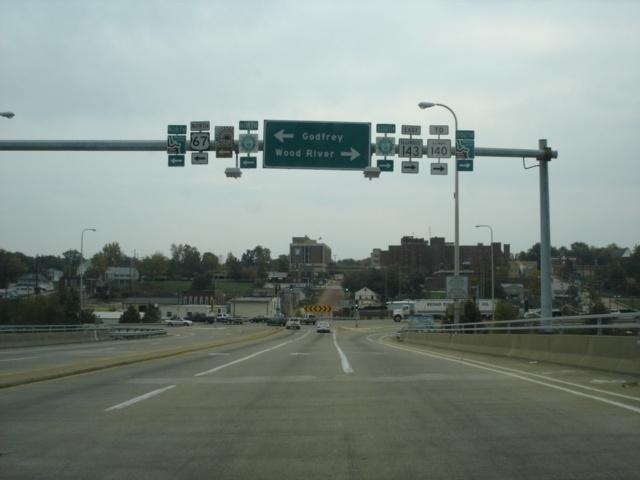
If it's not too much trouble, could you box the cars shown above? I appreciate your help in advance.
[248,315,301,330]
[216,313,243,325]
[317,322,329,332]
[166,317,192,326]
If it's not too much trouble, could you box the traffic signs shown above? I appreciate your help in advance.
[262,120,372,171]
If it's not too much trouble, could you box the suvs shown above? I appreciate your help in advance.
[302,314,316,325]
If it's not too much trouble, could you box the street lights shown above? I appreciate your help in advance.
[419,102,461,322]
[475,224,495,320]
[0,111,15,120]
[80,228,96,309]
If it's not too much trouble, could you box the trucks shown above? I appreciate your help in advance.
[392,299,496,322]
